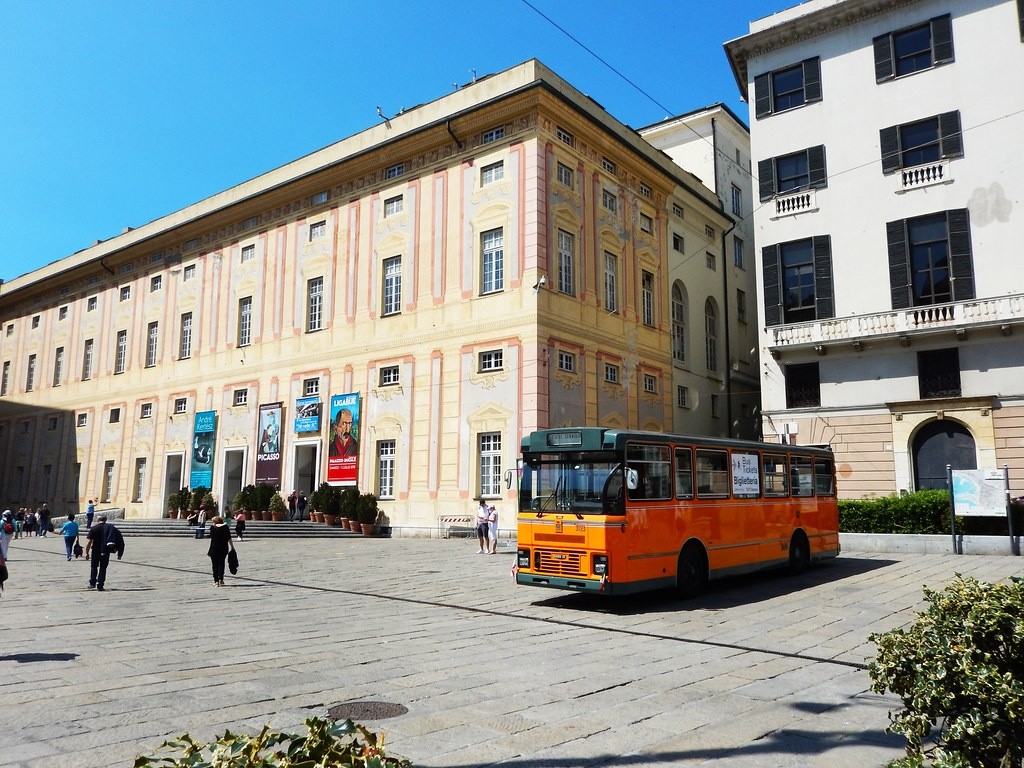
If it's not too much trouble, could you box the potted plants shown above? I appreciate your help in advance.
[165,480,378,536]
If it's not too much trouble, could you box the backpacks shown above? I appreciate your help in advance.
[2,519,13,535]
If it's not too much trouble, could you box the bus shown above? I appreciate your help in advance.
[503,427,843,602]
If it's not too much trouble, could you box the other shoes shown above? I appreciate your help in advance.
[40,535,47,538]
[87,585,95,588]
[213,582,218,587]
[237,538,242,541]
[67,557,71,561]
[489,551,496,555]
[219,580,224,584]
[97,588,105,591]
[485,549,490,554]
[476,550,484,554]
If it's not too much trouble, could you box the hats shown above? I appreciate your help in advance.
[2,510,11,515]
[225,506,229,509]
[480,499,485,506]
[200,505,205,508]
[486,504,495,509]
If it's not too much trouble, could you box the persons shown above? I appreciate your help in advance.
[59,514,79,561]
[194,436,212,463]
[187,510,198,525]
[12,503,51,540]
[476,499,490,554]
[222,505,232,527]
[198,505,206,539]
[483,504,498,554]
[234,509,247,541]
[85,514,114,591]
[0,510,18,561]
[0,541,8,584]
[206,517,234,588]
[289,490,298,521]
[296,491,307,522]
[300,404,319,418]
[86,498,98,529]
